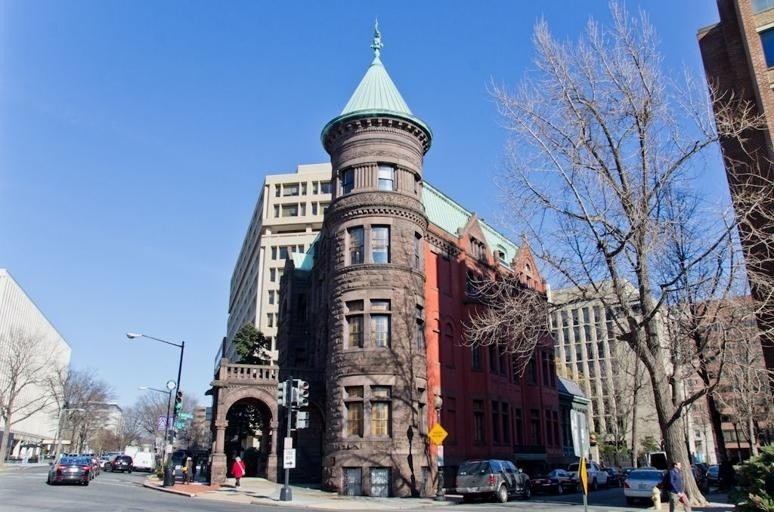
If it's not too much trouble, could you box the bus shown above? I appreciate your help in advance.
[173,449,208,466]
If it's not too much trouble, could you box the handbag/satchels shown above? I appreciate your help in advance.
[242,469,245,475]
[181,467,188,471]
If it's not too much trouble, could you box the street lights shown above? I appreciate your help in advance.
[126,332,184,463]
[55,408,84,440]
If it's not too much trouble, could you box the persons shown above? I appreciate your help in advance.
[666,461,691,512]
[232,457,246,488]
[181,451,197,485]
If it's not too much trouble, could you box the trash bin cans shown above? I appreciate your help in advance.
[164,467,175,486]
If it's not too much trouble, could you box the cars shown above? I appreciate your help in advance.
[456,451,719,502]
[46,452,155,485]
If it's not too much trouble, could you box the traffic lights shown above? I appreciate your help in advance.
[296,411,310,429]
[278,379,309,408]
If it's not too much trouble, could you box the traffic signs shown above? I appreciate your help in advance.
[283,448,296,469]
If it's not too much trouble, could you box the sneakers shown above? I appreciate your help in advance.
[235,485,241,489]
